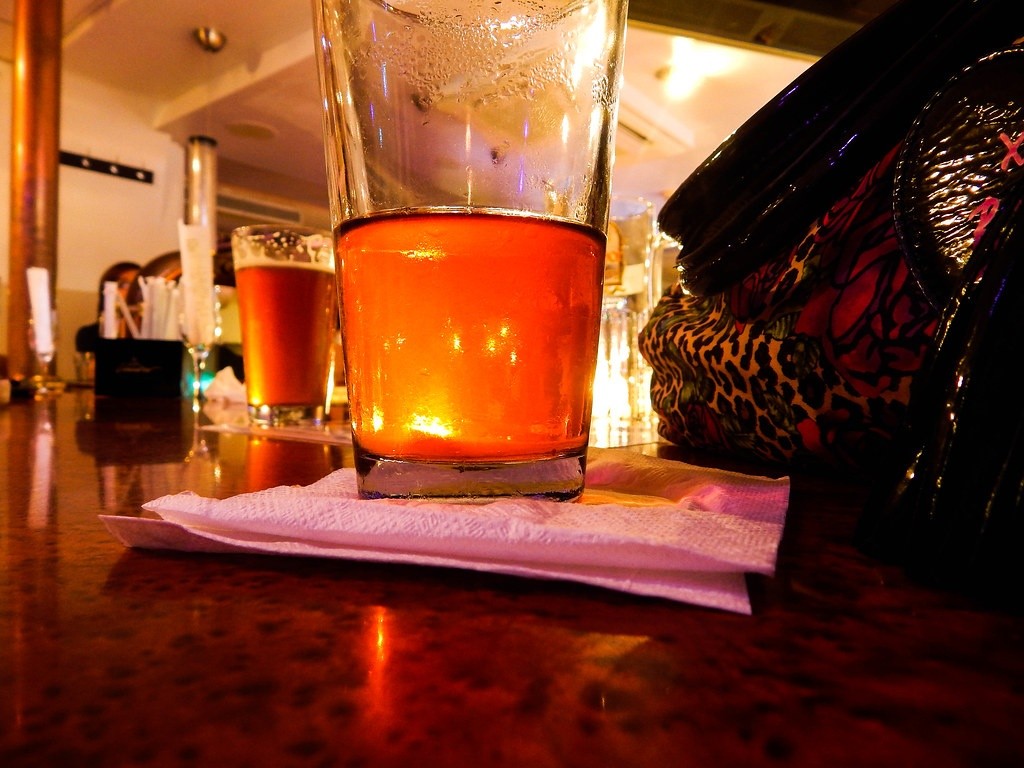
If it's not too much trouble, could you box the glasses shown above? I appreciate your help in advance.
[119,277,130,288]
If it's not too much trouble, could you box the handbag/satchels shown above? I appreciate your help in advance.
[638,1,1024,609]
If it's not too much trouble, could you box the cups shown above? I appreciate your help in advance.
[72,351,96,380]
[93,338,183,423]
[231,222,339,427]
[309,0,628,502]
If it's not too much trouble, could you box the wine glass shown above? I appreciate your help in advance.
[174,279,222,464]
[28,309,57,400]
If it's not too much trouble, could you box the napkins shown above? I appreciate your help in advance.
[99,446,790,616]
[25,267,54,354]
[177,219,216,343]
